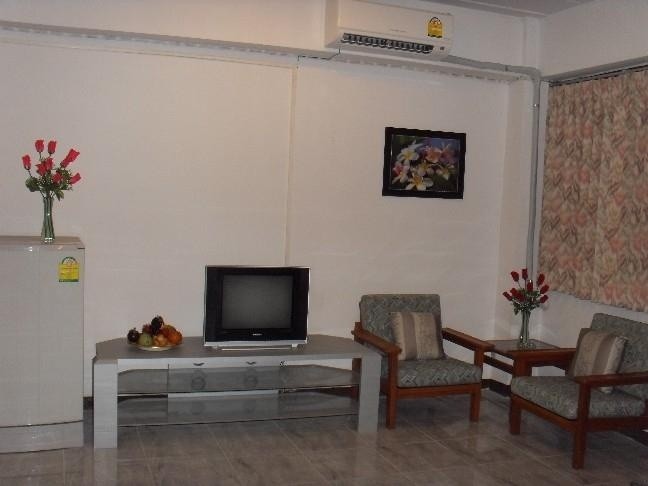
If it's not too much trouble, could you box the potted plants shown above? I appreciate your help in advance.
[380,125,466,200]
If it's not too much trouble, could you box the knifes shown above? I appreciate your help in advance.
[323,0,455,61]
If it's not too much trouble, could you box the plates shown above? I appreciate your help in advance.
[90,333,382,449]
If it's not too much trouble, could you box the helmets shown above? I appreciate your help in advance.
[130,343,181,353]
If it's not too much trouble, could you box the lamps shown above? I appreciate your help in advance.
[507,313,647,469]
[352,294,494,431]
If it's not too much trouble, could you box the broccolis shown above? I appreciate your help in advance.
[0,234,86,454]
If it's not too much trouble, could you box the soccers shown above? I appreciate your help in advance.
[139,333,152,346]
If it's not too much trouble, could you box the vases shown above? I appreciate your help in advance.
[502,267,549,312]
[20,137,83,241]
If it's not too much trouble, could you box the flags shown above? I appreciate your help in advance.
[516,308,533,347]
[39,195,55,242]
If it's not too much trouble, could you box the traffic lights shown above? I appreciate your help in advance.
[566,326,628,395]
[388,310,445,362]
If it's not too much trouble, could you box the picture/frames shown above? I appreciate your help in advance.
[478,339,561,402]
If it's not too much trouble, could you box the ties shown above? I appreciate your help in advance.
[203,265,308,350]
